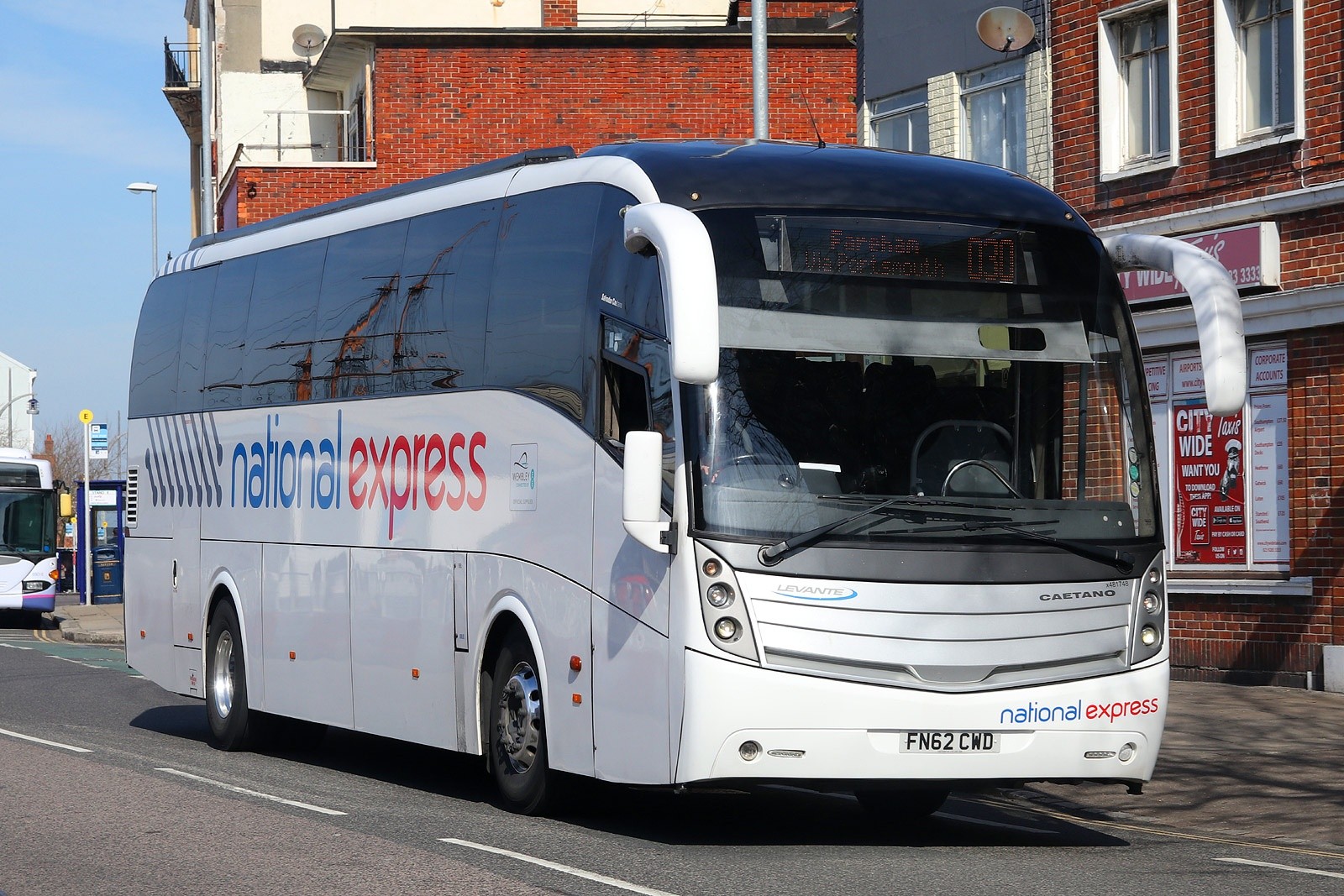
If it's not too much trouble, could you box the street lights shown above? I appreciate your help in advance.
[124,182,158,279]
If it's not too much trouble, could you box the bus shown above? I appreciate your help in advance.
[0,447,73,626]
[122,139,1247,820]
